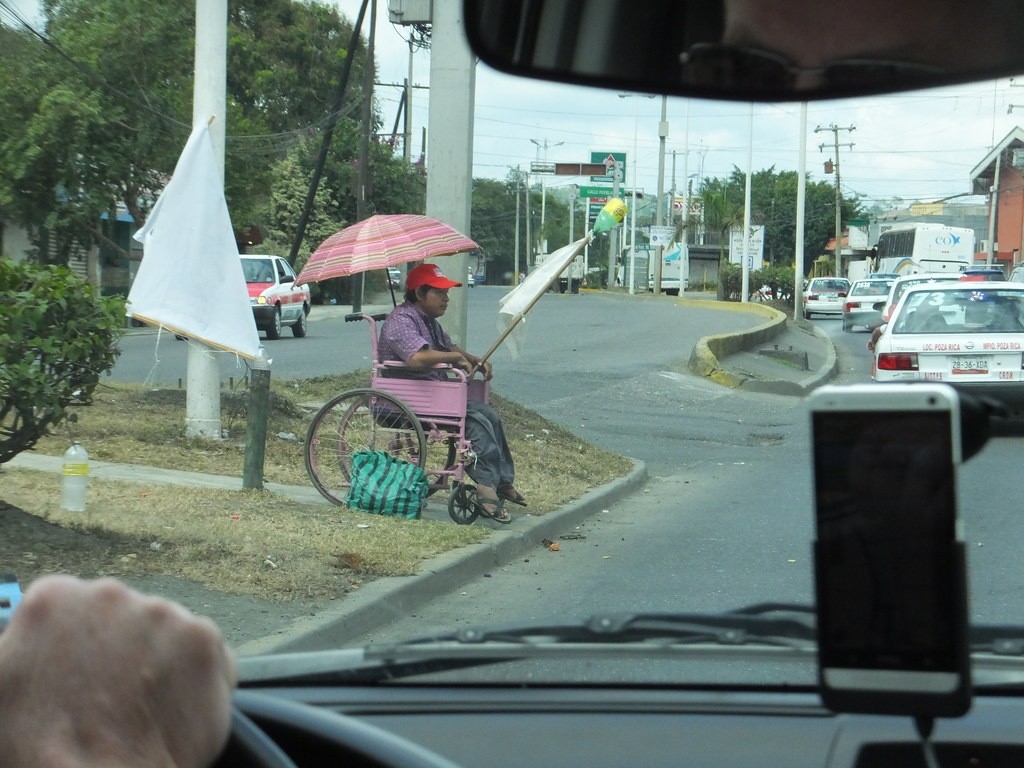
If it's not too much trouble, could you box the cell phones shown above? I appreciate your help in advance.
[807,383,963,695]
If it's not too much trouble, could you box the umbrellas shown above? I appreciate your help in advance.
[294,213,482,308]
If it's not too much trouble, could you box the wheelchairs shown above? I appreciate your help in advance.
[303,312,507,527]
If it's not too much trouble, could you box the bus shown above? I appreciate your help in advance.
[868,222,976,281]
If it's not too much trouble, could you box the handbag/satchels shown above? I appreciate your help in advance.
[344,449,425,520]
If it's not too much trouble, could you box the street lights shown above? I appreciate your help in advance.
[834,190,867,279]
[528,137,565,265]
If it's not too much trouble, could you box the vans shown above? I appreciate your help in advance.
[614,244,690,297]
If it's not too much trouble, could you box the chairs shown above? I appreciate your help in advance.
[907,310,947,331]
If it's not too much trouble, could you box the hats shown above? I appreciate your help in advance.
[407,263,462,292]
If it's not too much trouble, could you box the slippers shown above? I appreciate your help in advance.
[497,485,528,507]
[470,492,512,523]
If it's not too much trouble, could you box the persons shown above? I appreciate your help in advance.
[1,571,238,767]
[717,1,1023,91]
[377,263,528,523]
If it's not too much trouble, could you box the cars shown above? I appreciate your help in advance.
[468,266,475,287]
[803,277,852,319]
[837,278,897,333]
[386,266,401,289]
[762,261,1024,420]
[171,253,313,340]
